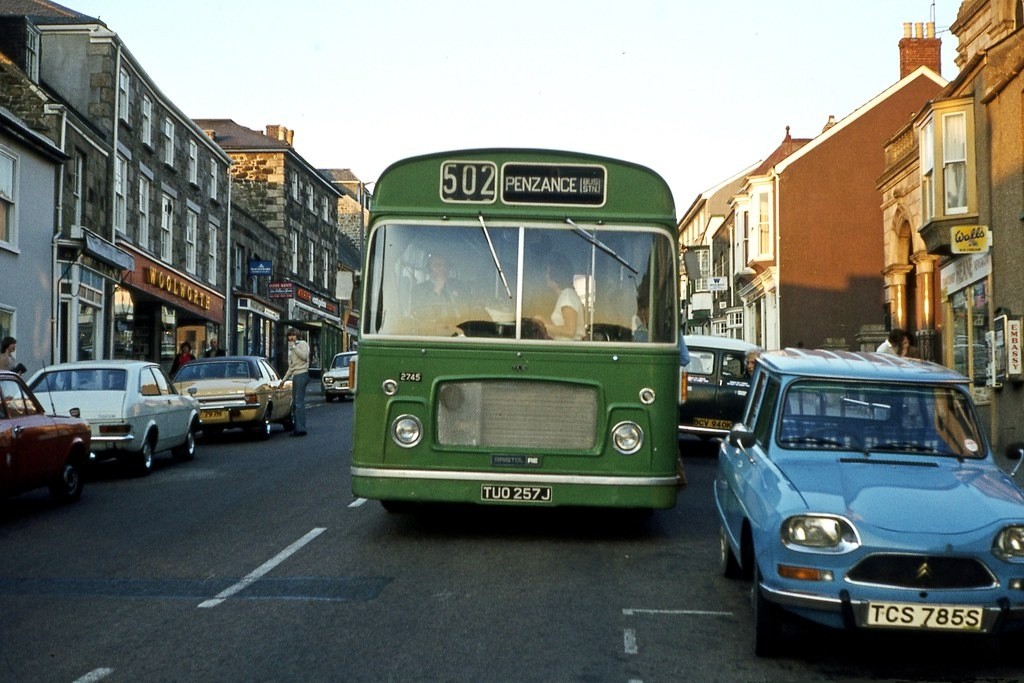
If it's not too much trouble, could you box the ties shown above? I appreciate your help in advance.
[442,289,456,324]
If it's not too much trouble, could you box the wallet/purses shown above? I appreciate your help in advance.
[11,363,27,374]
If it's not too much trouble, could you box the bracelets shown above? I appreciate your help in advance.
[292,345,295,348]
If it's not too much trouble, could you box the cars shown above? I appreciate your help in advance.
[172,355,294,440]
[8,357,202,478]
[679,333,765,441]
[0,369,94,507]
[713,348,1024,660]
[322,350,357,403]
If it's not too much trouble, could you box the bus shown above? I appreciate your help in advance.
[347,148,701,511]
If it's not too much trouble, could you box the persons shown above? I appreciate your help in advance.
[410,249,585,343]
[0,336,21,378]
[744,348,764,376]
[169,341,196,381]
[875,328,921,358]
[277,328,311,437]
[204,338,226,358]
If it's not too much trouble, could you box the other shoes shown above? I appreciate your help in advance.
[290,430,306,437]
